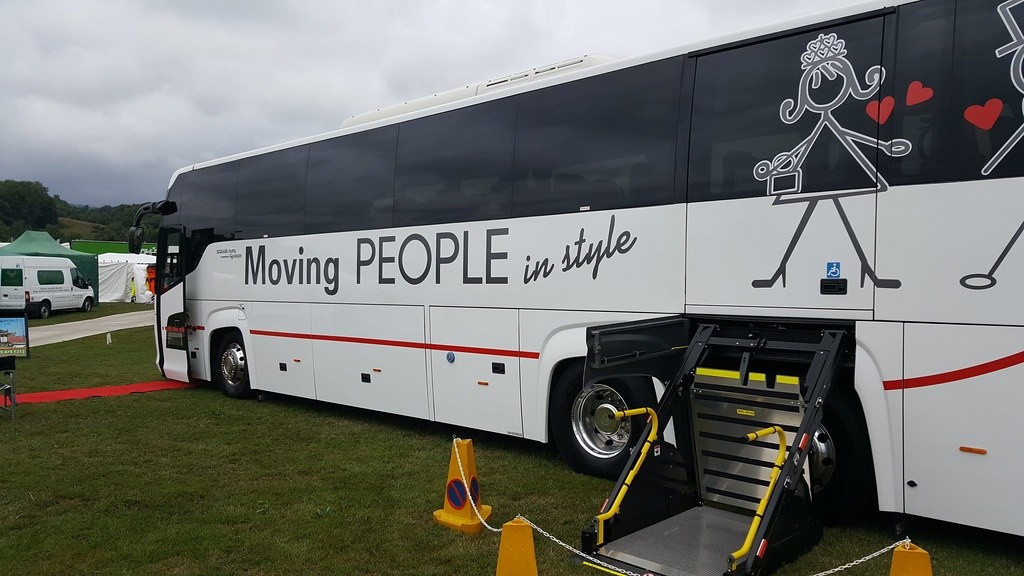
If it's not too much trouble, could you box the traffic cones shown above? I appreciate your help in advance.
[890,543,933,576]
[433,439,492,533]
[495,519,538,576]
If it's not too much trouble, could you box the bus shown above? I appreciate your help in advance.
[129,0,1024,540]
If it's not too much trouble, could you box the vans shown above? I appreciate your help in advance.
[0,255,95,319]
